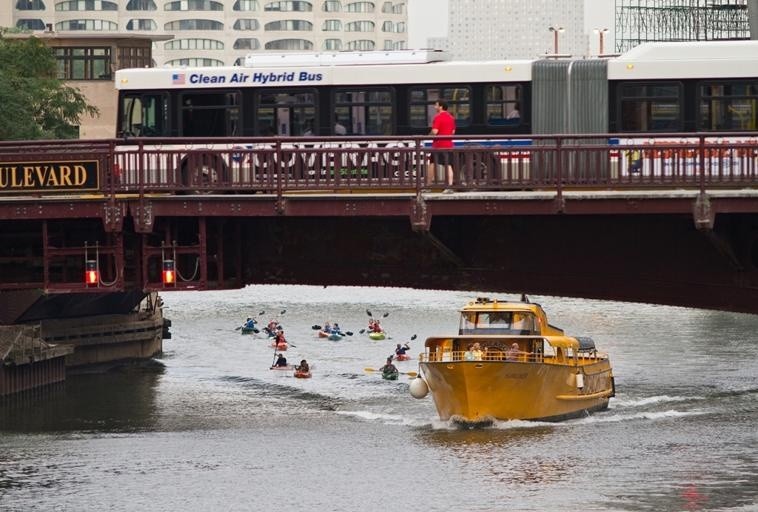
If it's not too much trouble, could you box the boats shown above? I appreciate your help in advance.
[240,326,412,379]
[420,297,614,428]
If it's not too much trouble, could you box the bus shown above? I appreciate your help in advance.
[114,39,756,193]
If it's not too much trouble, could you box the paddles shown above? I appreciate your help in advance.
[364,368,417,376]
[390,334,417,358]
[235,311,264,330]
[262,310,286,330]
[360,308,389,334]
[270,336,279,369]
[312,325,353,336]
[265,328,296,347]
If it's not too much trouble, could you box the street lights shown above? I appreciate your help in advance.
[550,27,564,53]
[593,27,609,53]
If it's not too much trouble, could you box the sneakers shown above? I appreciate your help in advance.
[421,188,453,194]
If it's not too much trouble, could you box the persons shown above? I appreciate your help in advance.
[335,113,346,136]
[419,101,457,194]
[264,126,278,137]
[463,344,476,360]
[245,314,409,374]
[472,343,489,361]
[507,343,532,360]
[491,315,506,324]
[506,102,519,120]
